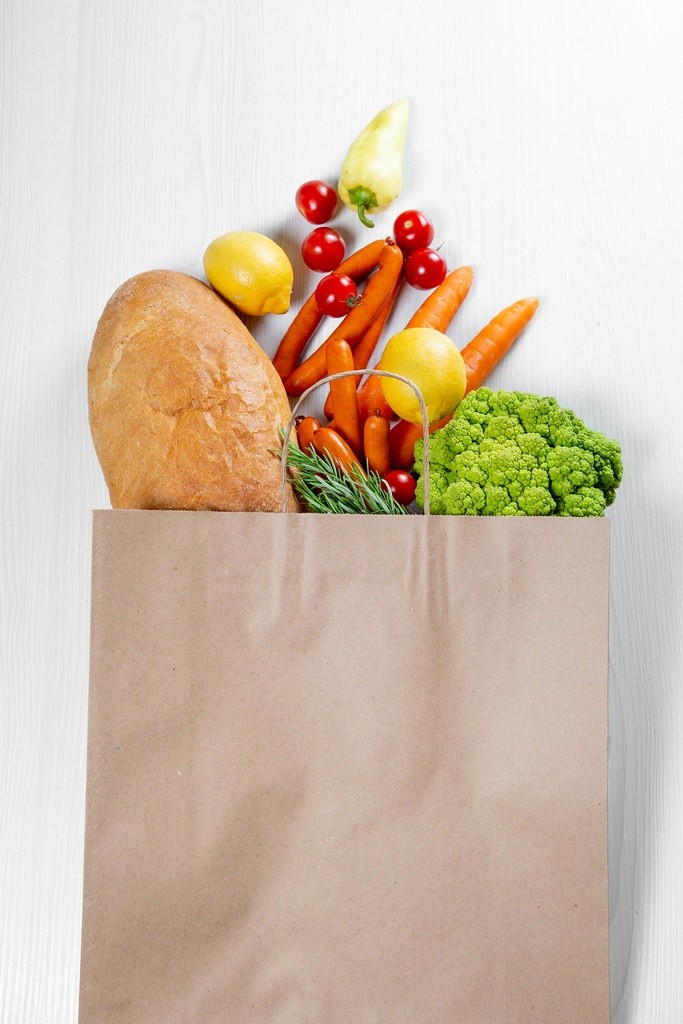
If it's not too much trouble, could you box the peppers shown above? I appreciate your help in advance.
[338,97,409,228]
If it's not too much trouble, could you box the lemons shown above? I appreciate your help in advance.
[203,231,294,316]
[380,327,467,428]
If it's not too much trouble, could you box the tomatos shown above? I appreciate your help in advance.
[403,248,447,291]
[294,179,338,225]
[393,209,434,252]
[302,226,345,273]
[315,273,357,318]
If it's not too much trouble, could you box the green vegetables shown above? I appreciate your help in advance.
[268,428,411,514]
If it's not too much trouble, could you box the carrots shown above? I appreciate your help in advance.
[389,296,539,470]
[324,264,473,440]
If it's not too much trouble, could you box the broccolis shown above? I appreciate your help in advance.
[412,387,623,517]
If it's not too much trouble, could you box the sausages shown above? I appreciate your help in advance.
[272,238,405,502]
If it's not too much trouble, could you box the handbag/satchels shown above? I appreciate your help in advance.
[77,369,612,1024]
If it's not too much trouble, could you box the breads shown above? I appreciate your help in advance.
[85,267,306,514]
